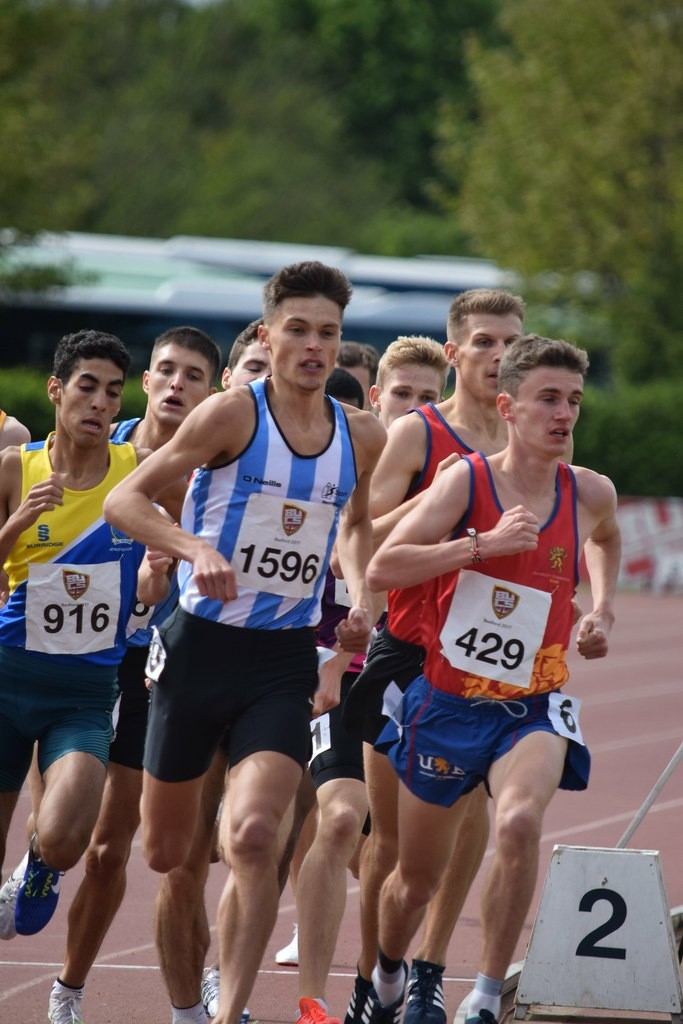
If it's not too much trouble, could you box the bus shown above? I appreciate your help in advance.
[1,221,534,389]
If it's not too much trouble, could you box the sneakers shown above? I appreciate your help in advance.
[403,967,446,1024]
[14,832,66,935]
[360,961,408,1024]
[199,967,250,1023]
[344,975,373,1024]
[295,998,342,1024]
[48,989,84,1024]
[0,849,30,941]
[465,1009,499,1024]
[275,923,298,966]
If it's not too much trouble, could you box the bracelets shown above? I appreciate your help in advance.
[469,536,480,562]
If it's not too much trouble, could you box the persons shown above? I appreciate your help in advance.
[361,331,621,1024]
[0,289,583,1024]
[102,263,389,1024]
